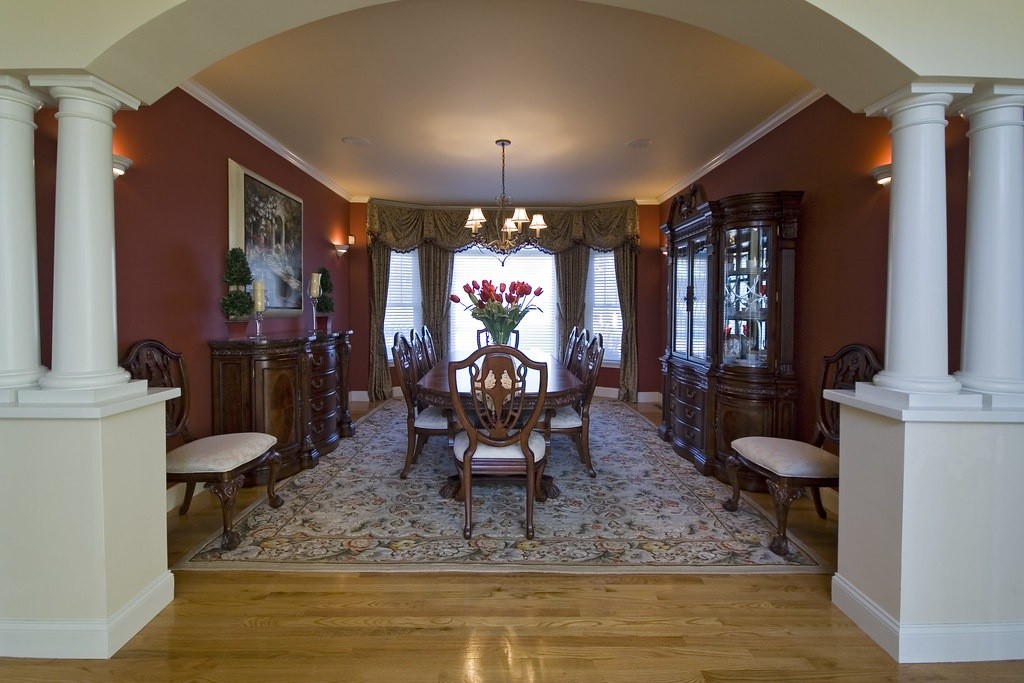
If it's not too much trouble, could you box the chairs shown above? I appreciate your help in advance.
[391,325,479,480]
[448,345,548,539]
[520,325,604,467]
[118,340,284,552]
[723,342,885,557]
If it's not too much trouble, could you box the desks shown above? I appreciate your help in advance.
[410,350,587,500]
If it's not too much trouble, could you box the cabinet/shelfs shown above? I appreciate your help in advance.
[660,183,806,493]
[209,330,353,488]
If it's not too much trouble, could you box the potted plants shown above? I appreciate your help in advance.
[316,267,335,334]
[221,248,255,341]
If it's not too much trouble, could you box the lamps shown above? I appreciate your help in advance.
[465,140,547,252]
[870,163,892,185]
[112,154,133,176]
[334,244,349,259]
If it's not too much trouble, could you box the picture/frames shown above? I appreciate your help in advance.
[228,157,303,321]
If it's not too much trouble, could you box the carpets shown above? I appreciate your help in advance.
[168,396,838,574]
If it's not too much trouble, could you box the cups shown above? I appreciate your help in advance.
[745,259,756,269]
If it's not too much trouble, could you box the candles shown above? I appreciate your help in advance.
[310,273,321,298]
[252,280,264,312]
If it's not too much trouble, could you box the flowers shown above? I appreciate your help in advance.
[449,280,545,346]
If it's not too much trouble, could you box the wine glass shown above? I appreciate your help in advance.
[744,337,756,353]
